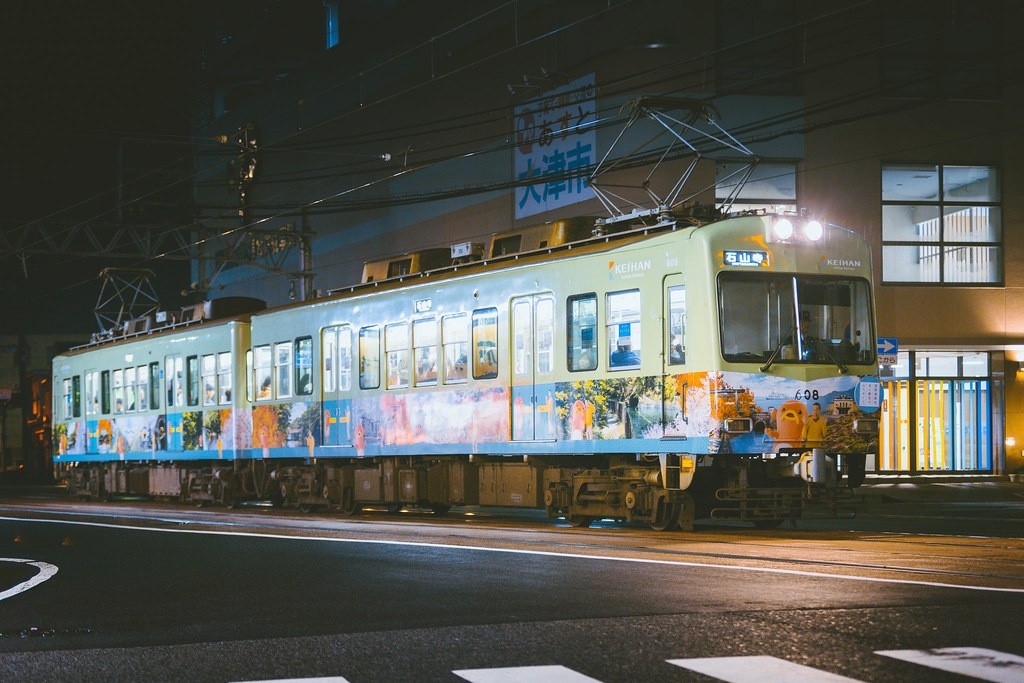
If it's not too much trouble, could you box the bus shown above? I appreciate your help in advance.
[30,208,883,535]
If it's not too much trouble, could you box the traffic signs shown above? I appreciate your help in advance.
[876,338,898,365]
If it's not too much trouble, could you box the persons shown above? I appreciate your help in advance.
[612,338,639,366]
[301,374,309,393]
[416,347,452,374]
[206,385,214,402]
[116,399,123,413]
[671,332,685,365]
[258,377,270,398]
[579,352,594,369]
[802,404,829,447]
[785,311,818,352]
[130,391,145,410]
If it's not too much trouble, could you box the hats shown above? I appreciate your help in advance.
[799,311,812,321]
